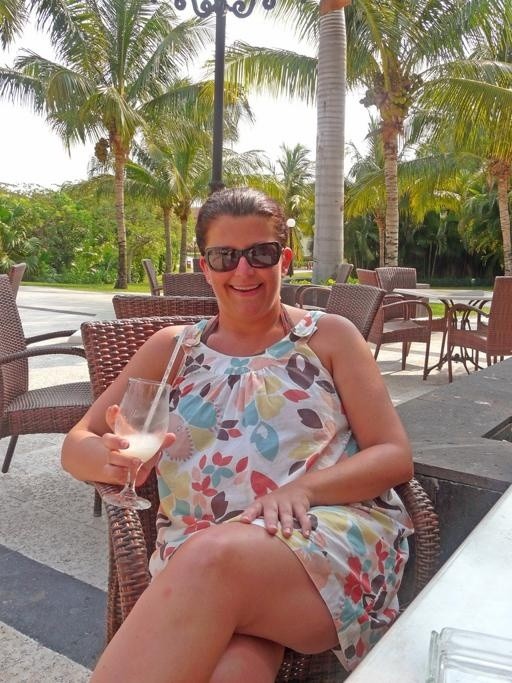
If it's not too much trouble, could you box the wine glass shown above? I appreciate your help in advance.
[102,378,173,511]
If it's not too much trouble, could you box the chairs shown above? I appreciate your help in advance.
[278,259,355,312]
[7,263,28,299]
[165,271,215,298]
[324,281,389,343]
[1,274,97,475]
[356,267,434,381]
[79,315,443,682]
[376,266,446,360]
[445,276,511,385]
[112,293,222,319]
[143,259,164,297]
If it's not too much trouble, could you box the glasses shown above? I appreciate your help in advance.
[201,241,282,272]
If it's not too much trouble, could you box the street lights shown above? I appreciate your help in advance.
[286,217,297,276]
[192,236,198,258]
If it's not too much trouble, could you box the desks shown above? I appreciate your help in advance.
[340,485,510,681]
[393,287,494,382]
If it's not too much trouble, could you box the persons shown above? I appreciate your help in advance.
[61,187,415,682]
